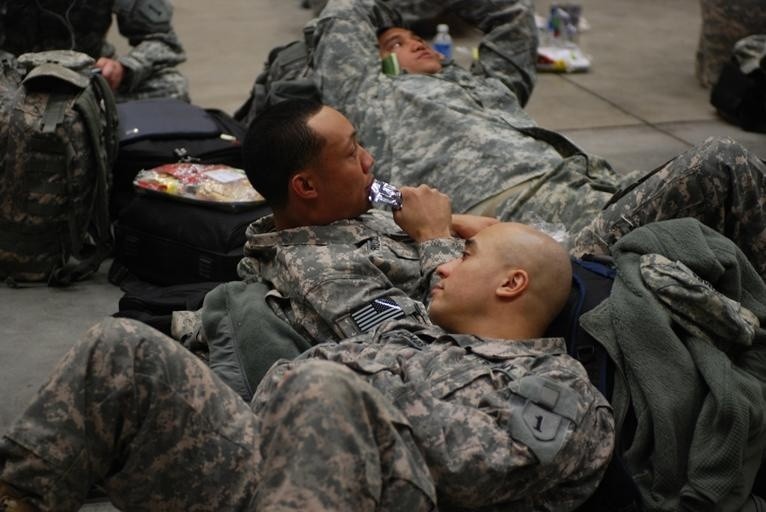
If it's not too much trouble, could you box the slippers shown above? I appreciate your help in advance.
[0,46,121,290]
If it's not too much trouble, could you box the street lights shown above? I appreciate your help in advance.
[709,34,766,136]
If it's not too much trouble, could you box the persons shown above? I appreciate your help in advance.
[0,0,194,104]
[302,1,766,269]
[698,2,766,93]
[0,220,615,512]
[231,79,498,356]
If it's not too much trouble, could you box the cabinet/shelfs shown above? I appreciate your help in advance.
[433,24,453,67]
